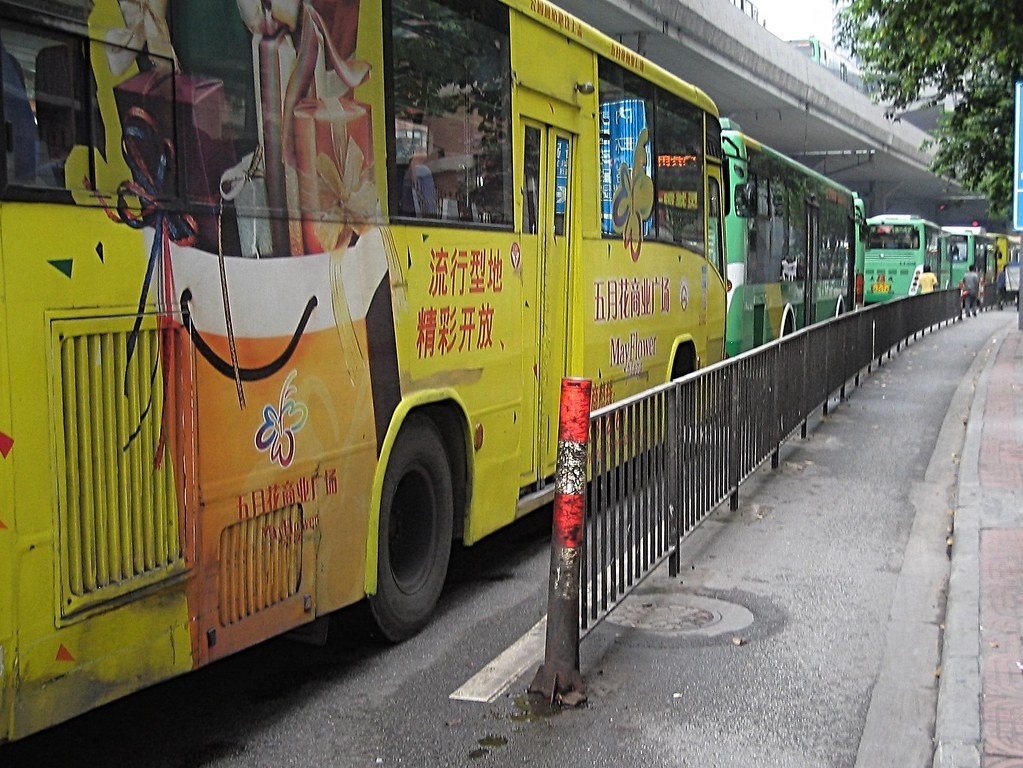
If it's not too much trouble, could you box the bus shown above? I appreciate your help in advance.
[0,1,755,746]
[940,225,1021,290]
[864,215,956,304]
[691,118,869,359]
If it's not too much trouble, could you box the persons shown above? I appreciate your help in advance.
[917,265,938,295]
[962,265,979,319]
[997,265,1010,311]
